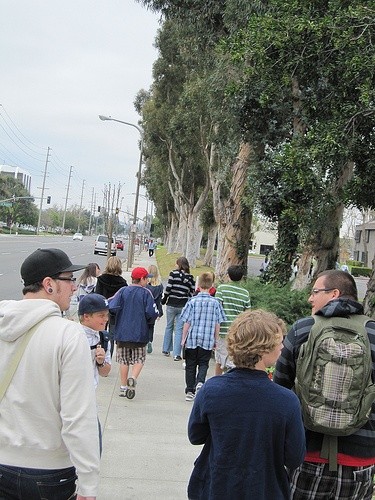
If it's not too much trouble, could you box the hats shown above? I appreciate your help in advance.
[21,248,87,286]
[78,294,120,314]
[131,268,153,279]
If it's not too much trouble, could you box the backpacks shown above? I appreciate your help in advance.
[295,314,375,471]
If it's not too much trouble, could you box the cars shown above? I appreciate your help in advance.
[72,233,83,240]
[116,240,125,251]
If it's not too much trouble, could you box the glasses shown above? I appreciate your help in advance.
[311,288,341,294]
[52,277,76,281]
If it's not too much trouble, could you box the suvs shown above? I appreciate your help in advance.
[93,235,117,256]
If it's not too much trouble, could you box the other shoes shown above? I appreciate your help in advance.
[147,342,152,353]
[119,386,128,397]
[162,352,170,357]
[173,356,181,361]
[185,392,195,401]
[196,383,203,392]
[127,378,135,399]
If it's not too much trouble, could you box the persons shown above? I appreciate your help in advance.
[155,241,158,248]
[272,269,375,500]
[77,263,101,302]
[144,239,151,252]
[0,247,102,500]
[187,309,306,500]
[107,267,159,399]
[261,257,269,276]
[146,265,163,354]
[215,264,251,378]
[97,256,128,356]
[149,240,155,257]
[77,293,120,387]
[160,257,195,362]
[195,272,216,297]
[179,272,227,401]
[340,263,348,271]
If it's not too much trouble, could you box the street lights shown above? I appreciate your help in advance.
[133,193,148,228]
[99,114,144,268]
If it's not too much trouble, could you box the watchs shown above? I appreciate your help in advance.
[96,361,105,366]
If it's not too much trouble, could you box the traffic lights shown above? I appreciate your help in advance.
[151,224,154,231]
[116,208,119,214]
